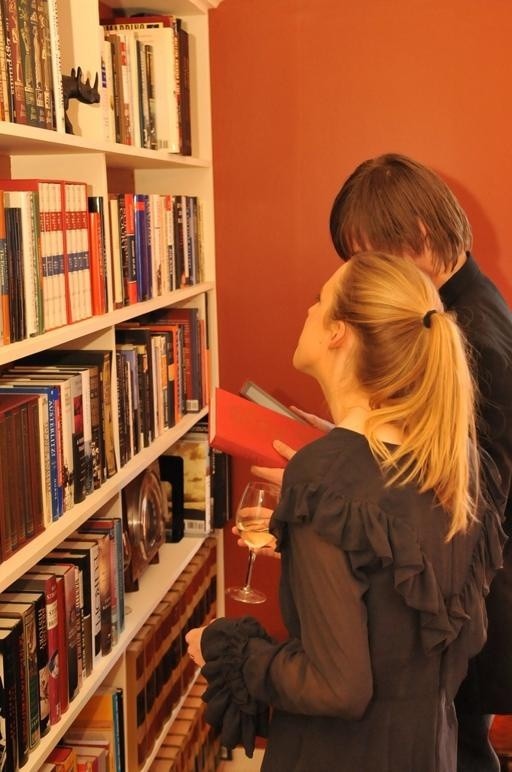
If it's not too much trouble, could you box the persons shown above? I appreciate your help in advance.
[248,152,511,772]
[184,250,496,772]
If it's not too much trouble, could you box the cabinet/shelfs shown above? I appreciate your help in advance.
[0,0,233,772]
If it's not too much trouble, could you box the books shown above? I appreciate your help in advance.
[37,686,126,771]
[126,537,218,771]
[160,423,232,537]
[108,192,205,311]
[210,387,328,470]
[99,2,193,156]
[1,178,109,346]
[115,307,211,468]
[148,673,221,772]
[1,345,117,562]
[0,2,67,135]
[0,516,125,771]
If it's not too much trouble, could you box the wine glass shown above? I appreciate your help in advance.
[223,482,282,607]
